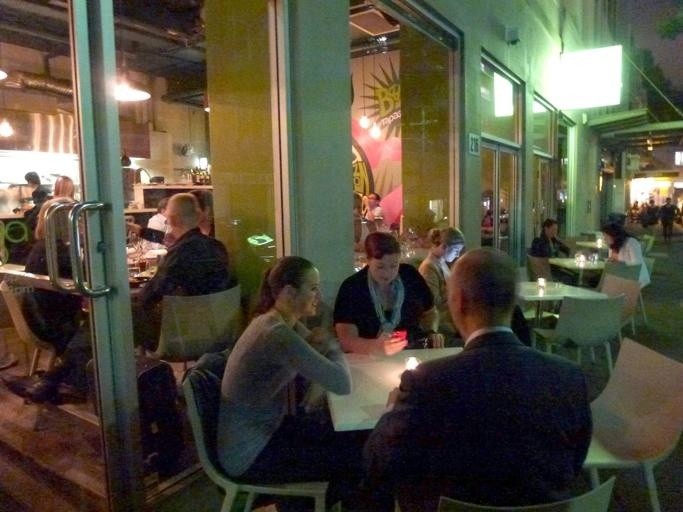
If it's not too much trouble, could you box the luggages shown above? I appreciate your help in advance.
[134,353,183,470]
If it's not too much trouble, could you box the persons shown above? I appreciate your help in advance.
[362,245,593,512]
[355,193,680,294]
[333,232,446,357]
[20,171,229,403]
[418,227,530,347]
[217,255,366,512]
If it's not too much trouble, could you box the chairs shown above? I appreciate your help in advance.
[530,294,624,377]
[2,280,58,432]
[507,217,681,322]
[437,476,615,511]
[144,284,242,382]
[583,338,682,511]
[183,372,331,510]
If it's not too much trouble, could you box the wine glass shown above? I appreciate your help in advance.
[126,229,142,267]
[408,227,419,256]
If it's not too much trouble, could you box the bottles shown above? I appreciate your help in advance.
[194,170,209,183]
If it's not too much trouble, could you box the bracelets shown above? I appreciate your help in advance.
[428,330,437,334]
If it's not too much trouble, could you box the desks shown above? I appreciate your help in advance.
[325,346,466,433]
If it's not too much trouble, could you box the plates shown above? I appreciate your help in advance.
[144,249,168,259]
[134,266,158,278]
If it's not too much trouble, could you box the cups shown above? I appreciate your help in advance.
[597,239,602,248]
[575,251,598,262]
[538,277,546,288]
[403,356,421,372]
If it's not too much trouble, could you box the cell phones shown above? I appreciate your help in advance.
[392,331,407,343]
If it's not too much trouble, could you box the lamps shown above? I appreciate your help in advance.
[367,50,383,141]
[110,0,152,102]
[356,40,369,129]
